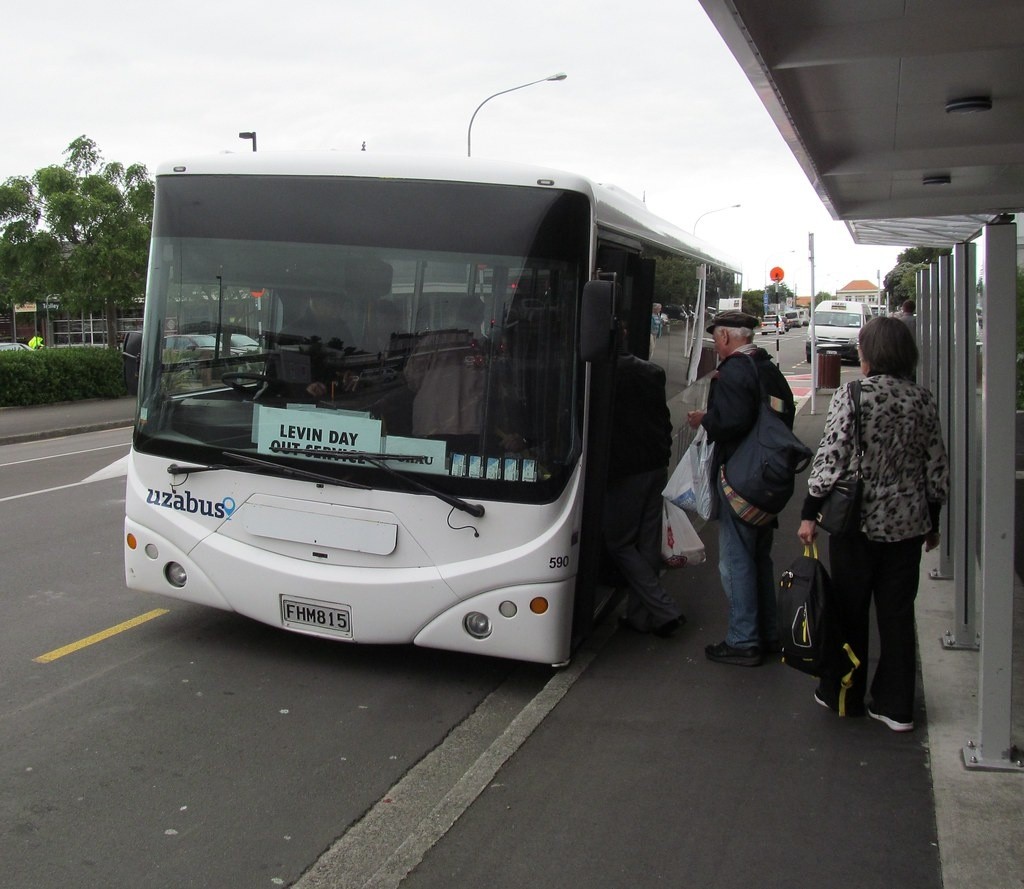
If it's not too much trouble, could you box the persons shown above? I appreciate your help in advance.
[898,300,915,341]
[403,296,485,448]
[28,331,44,350]
[265,292,362,402]
[500,323,687,638]
[687,310,796,666]
[649,303,662,359]
[798,317,949,731]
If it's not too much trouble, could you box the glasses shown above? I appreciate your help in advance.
[854,342,861,352]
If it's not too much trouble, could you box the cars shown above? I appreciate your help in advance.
[205,332,260,355]
[162,333,224,358]
[760,306,809,336]
[0,342,35,353]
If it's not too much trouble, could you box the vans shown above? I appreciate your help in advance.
[805,298,875,366]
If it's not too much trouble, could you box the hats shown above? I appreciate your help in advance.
[703,308,760,331]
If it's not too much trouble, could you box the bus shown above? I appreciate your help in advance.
[116,148,746,676]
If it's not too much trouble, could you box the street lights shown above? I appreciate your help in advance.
[693,204,742,238]
[466,70,568,158]
[211,276,223,379]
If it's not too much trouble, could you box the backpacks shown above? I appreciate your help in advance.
[770,537,862,716]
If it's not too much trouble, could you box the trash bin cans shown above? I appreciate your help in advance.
[816,343,842,389]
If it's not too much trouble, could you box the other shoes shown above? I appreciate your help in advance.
[813,688,829,708]
[617,615,649,634]
[867,699,916,732]
[650,614,690,639]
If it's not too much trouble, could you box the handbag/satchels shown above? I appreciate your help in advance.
[659,497,709,566]
[715,401,813,530]
[658,425,701,515]
[689,427,723,521]
[819,479,883,542]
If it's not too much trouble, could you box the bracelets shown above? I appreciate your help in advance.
[523,438,526,444]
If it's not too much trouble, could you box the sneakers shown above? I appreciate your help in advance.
[760,641,782,654]
[705,640,763,668]
